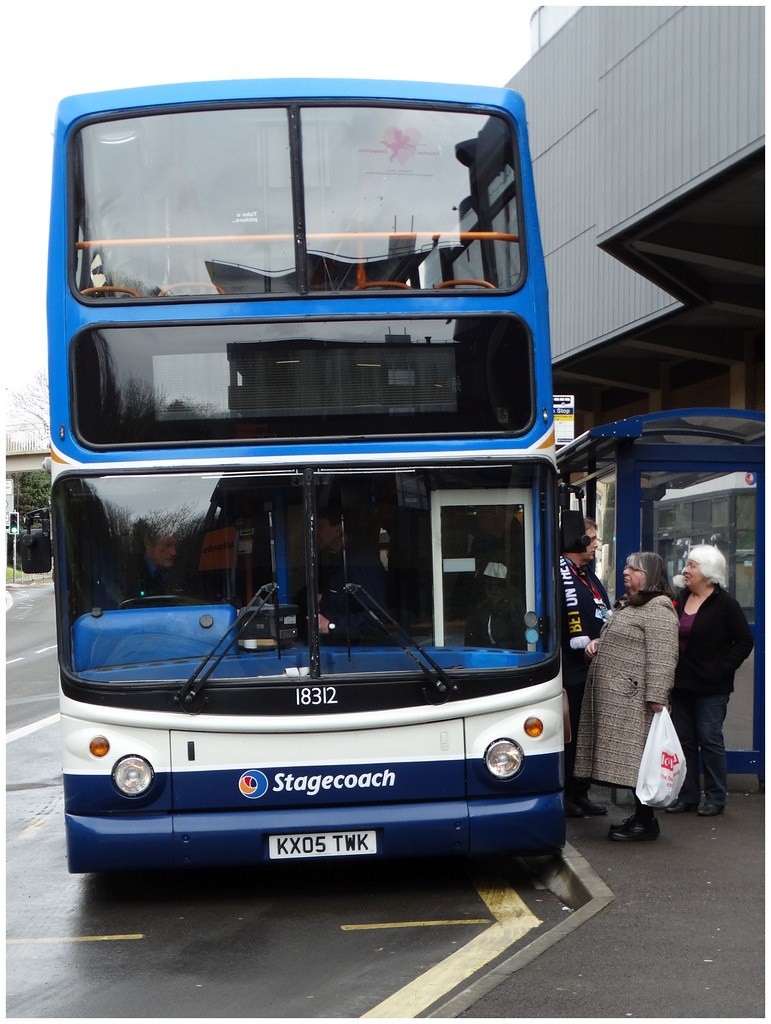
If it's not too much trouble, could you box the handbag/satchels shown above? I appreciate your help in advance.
[634,705,687,808]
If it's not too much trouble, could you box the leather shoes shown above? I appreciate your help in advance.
[609,816,660,842]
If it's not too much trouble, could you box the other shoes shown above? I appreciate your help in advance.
[697,803,725,817]
[665,801,698,812]
[564,799,584,818]
[576,796,608,815]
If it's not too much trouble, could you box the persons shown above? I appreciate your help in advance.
[561,518,753,843]
[104,487,525,651]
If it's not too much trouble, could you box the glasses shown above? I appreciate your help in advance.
[625,565,647,574]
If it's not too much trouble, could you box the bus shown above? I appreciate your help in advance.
[18,77,590,874]
